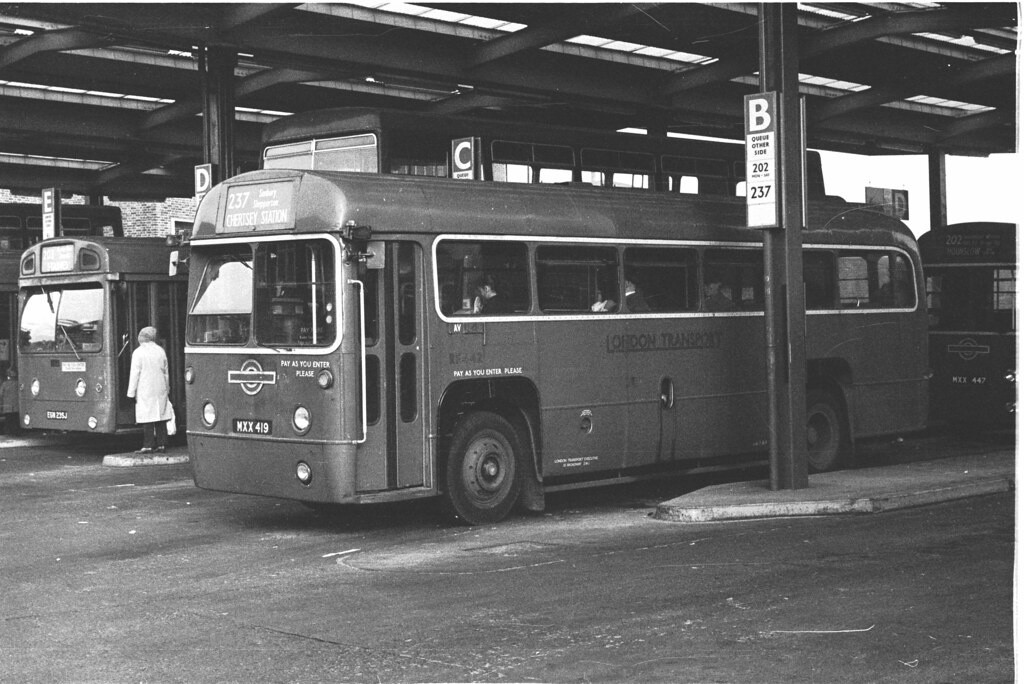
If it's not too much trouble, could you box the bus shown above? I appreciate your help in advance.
[916,223,1016,443]
[170,169,932,529]
[14,232,189,445]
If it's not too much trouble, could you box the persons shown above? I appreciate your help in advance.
[127,327,177,455]
[474,275,517,318]
[692,272,741,311]
[592,272,651,314]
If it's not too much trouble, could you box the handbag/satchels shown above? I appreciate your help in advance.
[165,399,177,436]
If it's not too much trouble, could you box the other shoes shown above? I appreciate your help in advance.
[154,445,165,453]
[134,448,153,454]
